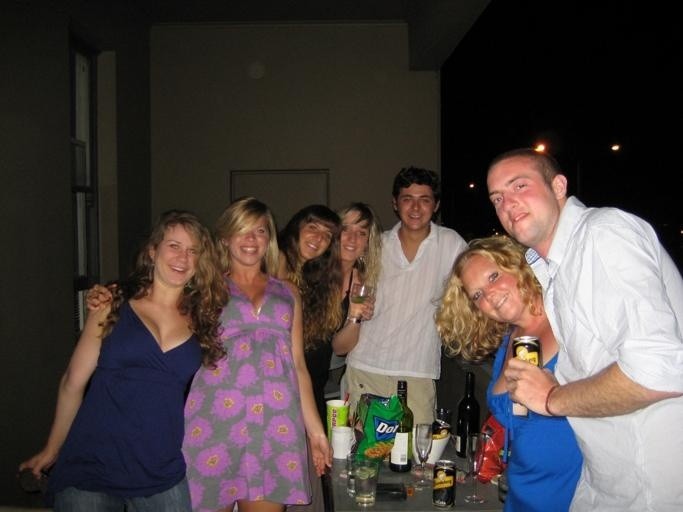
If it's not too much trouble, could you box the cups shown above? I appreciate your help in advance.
[327,401,383,506]
[432,409,453,426]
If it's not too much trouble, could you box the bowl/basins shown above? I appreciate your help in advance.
[408,428,450,465]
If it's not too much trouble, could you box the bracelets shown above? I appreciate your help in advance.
[347,316,362,324]
[544,385,559,418]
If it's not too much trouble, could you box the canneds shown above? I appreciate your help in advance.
[512,335,542,369]
[433,459,457,509]
[425,419,453,440]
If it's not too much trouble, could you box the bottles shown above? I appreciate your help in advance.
[456,371,481,458]
[498,447,513,503]
[389,380,415,472]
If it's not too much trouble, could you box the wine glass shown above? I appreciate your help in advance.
[412,424,433,488]
[465,433,486,503]
[346,282,376,324]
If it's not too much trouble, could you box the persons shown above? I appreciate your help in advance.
[340,165,470,436]
[260,205,340,351]
[304,202,382,437]
[85,197,334,512]
[488,146,683,512]
[433,234,583,511]
[16,211,231,512]
